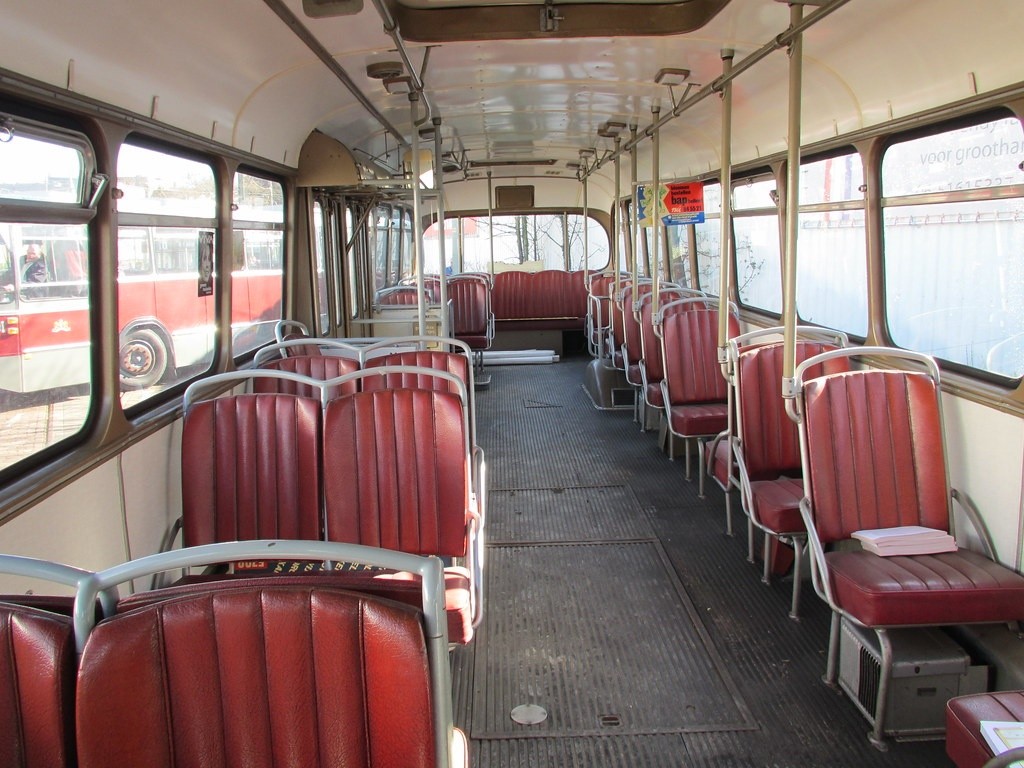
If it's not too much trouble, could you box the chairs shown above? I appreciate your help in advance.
[586,272,1023,767]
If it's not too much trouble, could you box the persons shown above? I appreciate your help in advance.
[446,258,452,274]
[198,234,213,296]
[0,243,47,298]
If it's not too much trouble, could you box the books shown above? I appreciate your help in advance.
[979,720,1024,768]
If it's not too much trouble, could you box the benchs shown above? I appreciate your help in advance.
[0,274,492,768]
[416,270,603,334]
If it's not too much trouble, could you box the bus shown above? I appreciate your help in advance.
[0,183,477,395]
